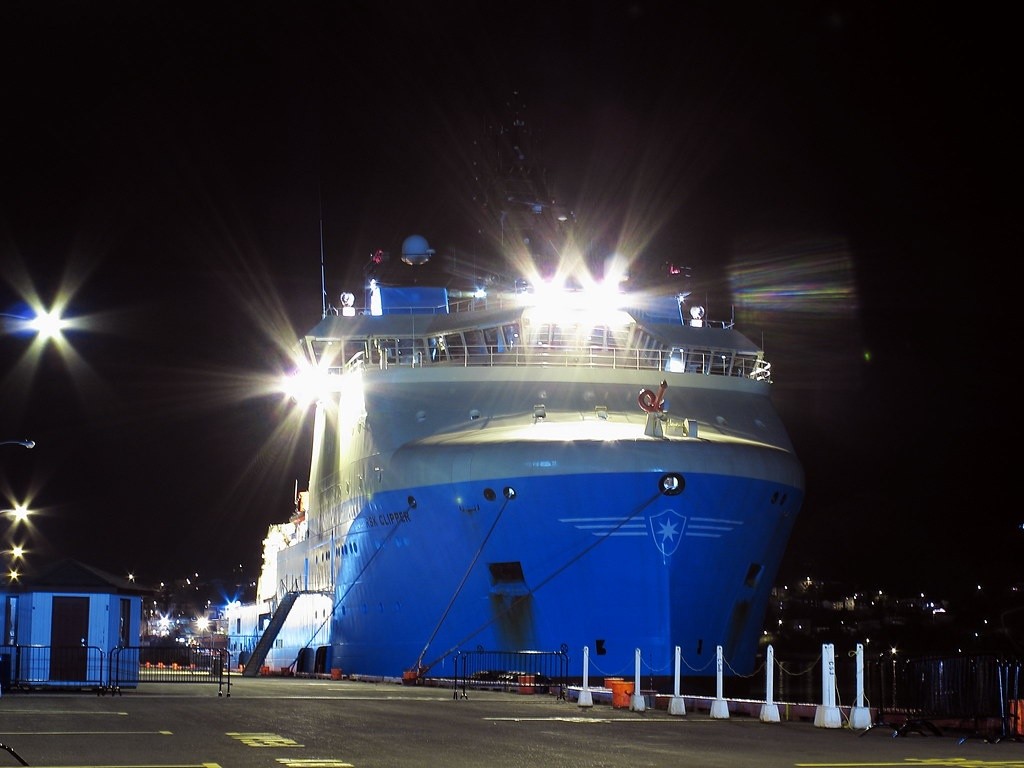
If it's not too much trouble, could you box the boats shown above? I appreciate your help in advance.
[288,511,305,524]
[226,207,809,700]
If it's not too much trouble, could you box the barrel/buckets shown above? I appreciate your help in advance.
[604,677,624,689]
[331,668,342,679]
[612,681,634,709]
